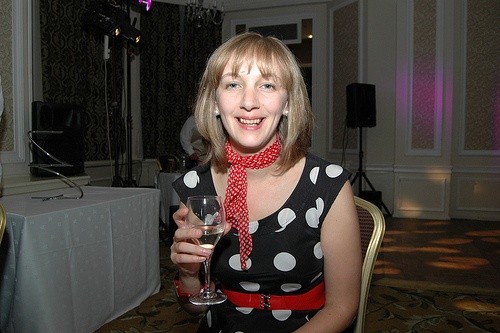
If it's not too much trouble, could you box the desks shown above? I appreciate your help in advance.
[0,186,161,333]
[157,172,187,226]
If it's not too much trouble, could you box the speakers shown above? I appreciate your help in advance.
[346,83,376,128]
[31,101,85,178]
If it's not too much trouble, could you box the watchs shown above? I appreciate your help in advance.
[172,271,206,303]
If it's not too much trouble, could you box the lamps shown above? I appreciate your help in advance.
[187,0,225,28]
[81,3,142,45]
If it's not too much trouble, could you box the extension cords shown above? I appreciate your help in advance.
[103,35,110,60]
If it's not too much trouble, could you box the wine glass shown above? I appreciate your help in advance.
[185,196,227,305]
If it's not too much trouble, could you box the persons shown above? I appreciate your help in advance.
[180,114,211,169]
[169,32,363,333]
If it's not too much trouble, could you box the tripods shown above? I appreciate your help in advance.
[351,128,392,217]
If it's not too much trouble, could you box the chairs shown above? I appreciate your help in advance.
[343,196,387,333]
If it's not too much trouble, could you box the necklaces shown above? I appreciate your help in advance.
[215,131,282,271]
